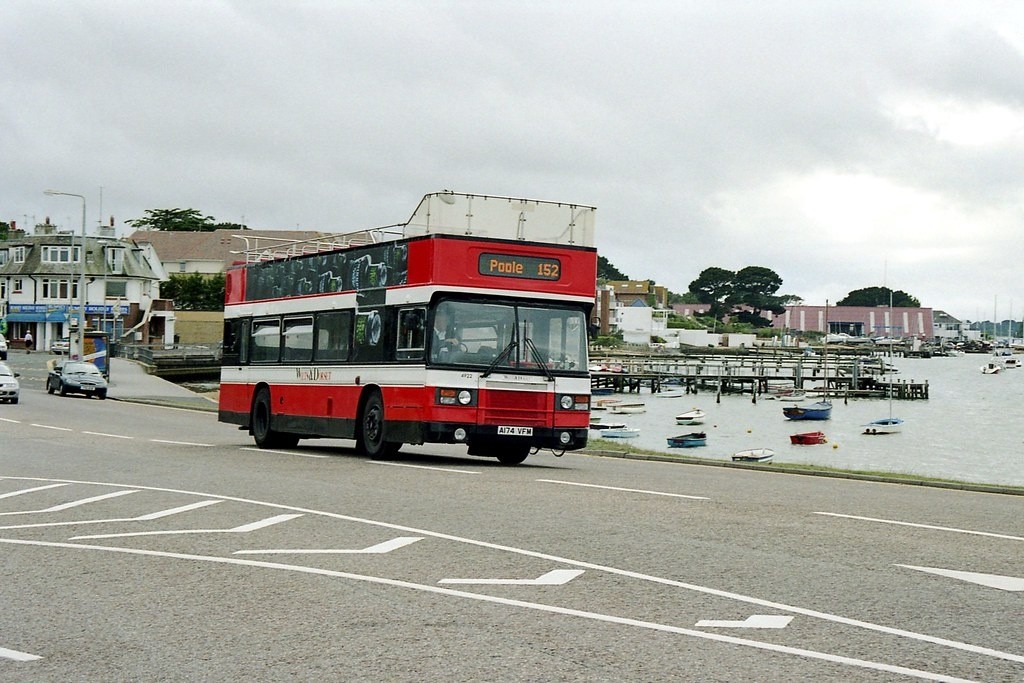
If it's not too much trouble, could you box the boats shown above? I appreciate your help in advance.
[600,427,641,438]
[590,386,615,395]
[655,387,687,397]
[666,432,708,448]
[860,361,901,375]
[590,421,627,430]
[730,446,775,467]
[790,431,826,445]
[774,387,808,403]
[606,402,647,415]
[591,398,622,410]
[675,408,709,425]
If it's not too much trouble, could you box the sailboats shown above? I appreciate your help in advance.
[858,292,905,435]
[782,298,834,421]
[1002,299,1022,369]
[980,295,1000,375]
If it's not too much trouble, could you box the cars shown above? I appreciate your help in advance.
[0,332,11,360]
[50,336,73,355]
[44,360,108,401]
[0,362,22,404]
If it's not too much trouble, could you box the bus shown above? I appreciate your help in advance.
[217,190,599,462]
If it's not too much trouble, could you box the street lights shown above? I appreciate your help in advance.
[96,238,110,332]
[44,189,88,362]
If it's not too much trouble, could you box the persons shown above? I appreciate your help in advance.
[430,310,460,362]
[24,329,34,354]
